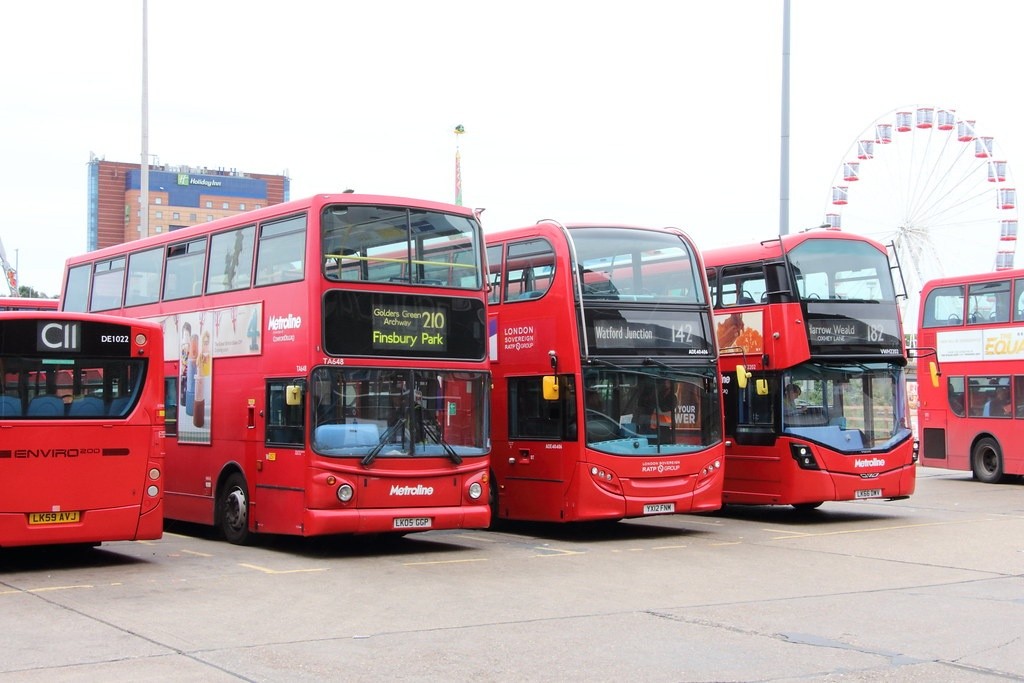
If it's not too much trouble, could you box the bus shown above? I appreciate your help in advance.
[1,308,168,549]
[490,223,944,510]
[917,266,1024,484]
[258,218,752,524]
[57,190,492,548]
[1,295,59,382]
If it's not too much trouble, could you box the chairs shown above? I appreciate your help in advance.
[0,393,23,415]
[736,290,756,304]
[948,310,1024,326]
[67,396,105,416]
[760,291,767,302]
[983,401,998,416]
[26,395,65,416]
[107,398,130,416]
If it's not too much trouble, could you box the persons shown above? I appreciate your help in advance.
[772,383,808,423]
[949,380,1010,416]
